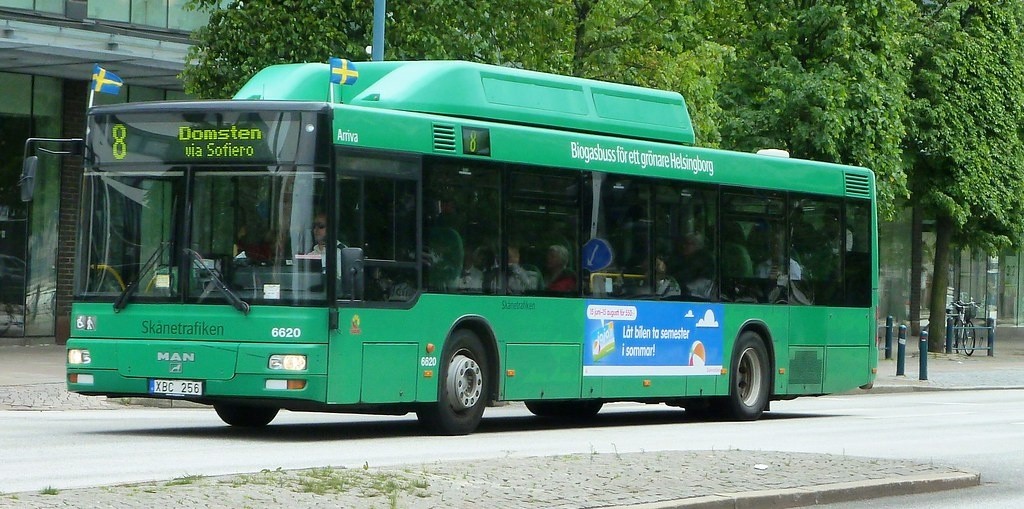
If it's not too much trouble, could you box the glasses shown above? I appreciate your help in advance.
[311,221,327,229]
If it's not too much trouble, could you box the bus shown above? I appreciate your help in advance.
[18,53,883,440]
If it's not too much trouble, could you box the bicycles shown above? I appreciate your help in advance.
[948,299,984,355]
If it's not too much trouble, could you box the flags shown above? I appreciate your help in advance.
[329,57,359,85]
[90,65,124,95]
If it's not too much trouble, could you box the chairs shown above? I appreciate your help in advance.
[429,225,801,301]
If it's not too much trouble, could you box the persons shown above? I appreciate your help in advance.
[544,243,580,294]
[484,238,537,294]
[630,252,681,297]
[753,235,802,282]
[309,213,348,281]
[818,207,854,255]
